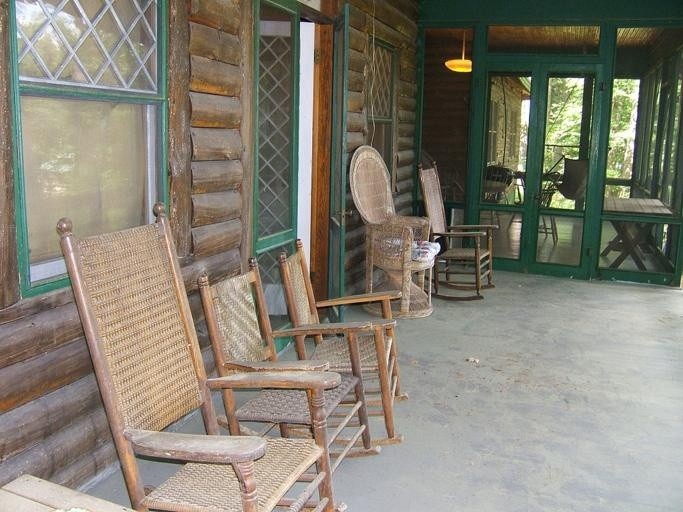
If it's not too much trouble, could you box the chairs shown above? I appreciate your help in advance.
[417,160,500,301]
[56,202,347,512]
[197,257,381,482]
[278,238,409,446]
[439,165,561,245]
[349,145,434,319]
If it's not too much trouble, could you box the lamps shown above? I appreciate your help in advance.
[445,27,473,72]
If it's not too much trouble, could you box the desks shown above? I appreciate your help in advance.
[603,197,673,271]
[0,473,125,507]
